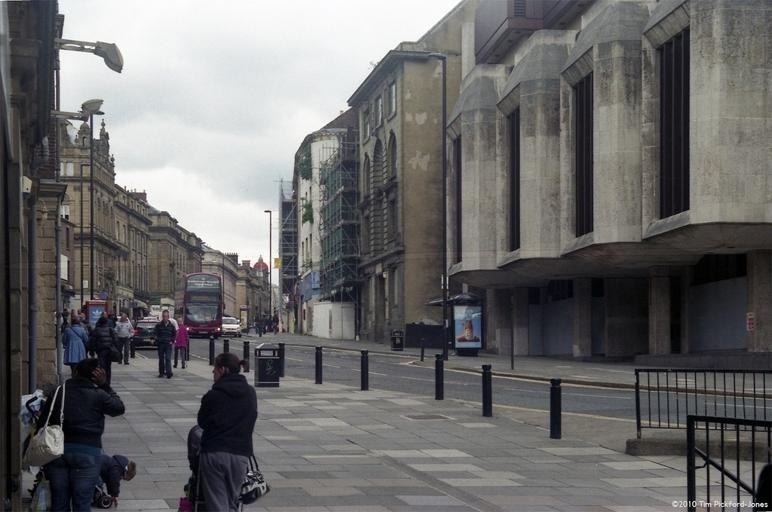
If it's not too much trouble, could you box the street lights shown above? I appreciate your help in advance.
[89,108,105,300]
[264,209,272,332]
[427,51,449,360]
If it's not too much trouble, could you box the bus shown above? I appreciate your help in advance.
[182,272,226,340]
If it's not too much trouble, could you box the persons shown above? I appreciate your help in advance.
[33,358,126,511]
[98,453,136,507]
[252,314,280,337]
[197,353,259,511]
[59,306,190,384]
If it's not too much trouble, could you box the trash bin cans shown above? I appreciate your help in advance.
[255,343,279,388]
[391,330,403,351]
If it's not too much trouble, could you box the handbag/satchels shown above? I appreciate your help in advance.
[107,344,122,362]
[241,455,269,504]
[28,478,52,512]
[24,424,64,466]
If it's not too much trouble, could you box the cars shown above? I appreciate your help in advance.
[222,315,242,338]
[132,317,161,348]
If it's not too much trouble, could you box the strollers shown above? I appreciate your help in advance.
[25,395,115,509]
